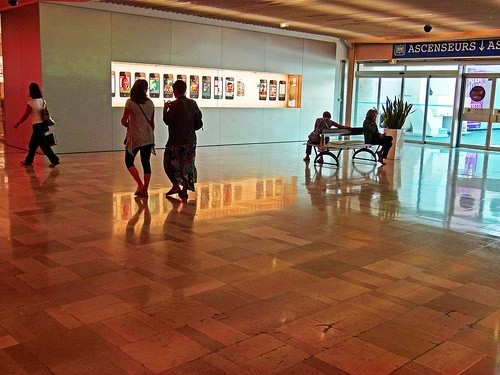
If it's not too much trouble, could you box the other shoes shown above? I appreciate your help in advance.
[377,151,382,157]
[49,161,60,166]
[303,158,310,161]
[316,159,323,163]
[179,191,189,199]
[166,188,181,194]
[378,159,386,165]
[20,160,32,165]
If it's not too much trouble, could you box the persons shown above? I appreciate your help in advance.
[163,80,203,198]
[14,82,61,167]
[121,80,156,195]
[303,111,350,162]
[363,109,393,164]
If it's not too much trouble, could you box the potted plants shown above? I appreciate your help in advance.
[380,95,413,160]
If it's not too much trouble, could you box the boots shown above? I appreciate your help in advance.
[129,166,145,194]
[139,174,151,195]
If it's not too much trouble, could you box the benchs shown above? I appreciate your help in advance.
[303,127,380,166]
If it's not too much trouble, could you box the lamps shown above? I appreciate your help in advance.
[424,20,431,32]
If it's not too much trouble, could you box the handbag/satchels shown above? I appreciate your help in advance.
[41,99,55,126]
[45,133,58,146]
